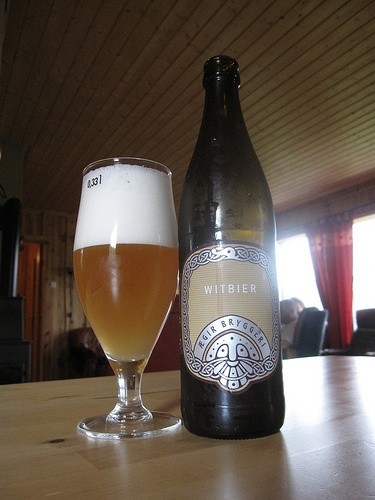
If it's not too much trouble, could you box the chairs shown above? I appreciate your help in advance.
[293,307,375,357]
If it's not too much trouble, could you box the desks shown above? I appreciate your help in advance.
[0,353,375,500]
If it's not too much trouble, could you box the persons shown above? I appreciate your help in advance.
[280,297,306,359]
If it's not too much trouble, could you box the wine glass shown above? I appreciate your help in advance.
[73,157,182,440]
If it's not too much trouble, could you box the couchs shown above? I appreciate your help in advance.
[65,327,116,377]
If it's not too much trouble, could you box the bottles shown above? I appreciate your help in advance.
[179,54,285,440]
